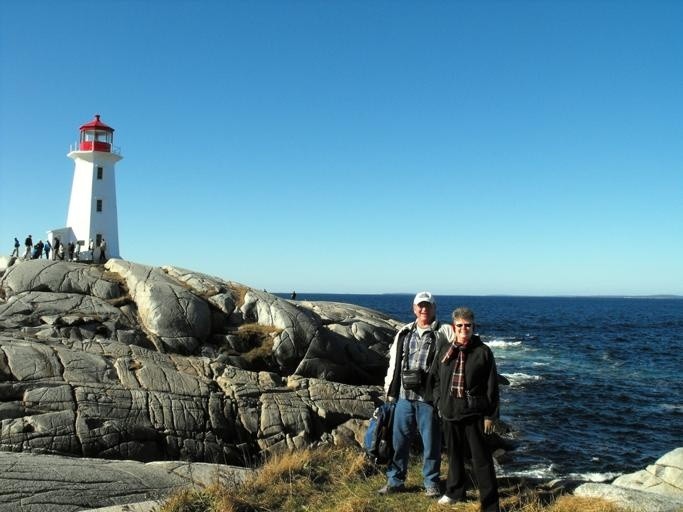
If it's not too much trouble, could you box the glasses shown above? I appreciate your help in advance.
[456,324,471,327]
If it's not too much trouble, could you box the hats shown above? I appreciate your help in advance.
[413,292,436,305]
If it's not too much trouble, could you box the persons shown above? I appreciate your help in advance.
[10,235,80,261]
[377,292,456,496]
[89,239,94,260]
[425,306,500,512]
[99,239,106,260]
[290,291,296,299]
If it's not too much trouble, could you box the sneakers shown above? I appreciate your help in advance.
[376,484,406,494]
[438,494,459,505]
[426,486,440,496]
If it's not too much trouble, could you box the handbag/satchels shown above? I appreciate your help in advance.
[365,403,395,465]
